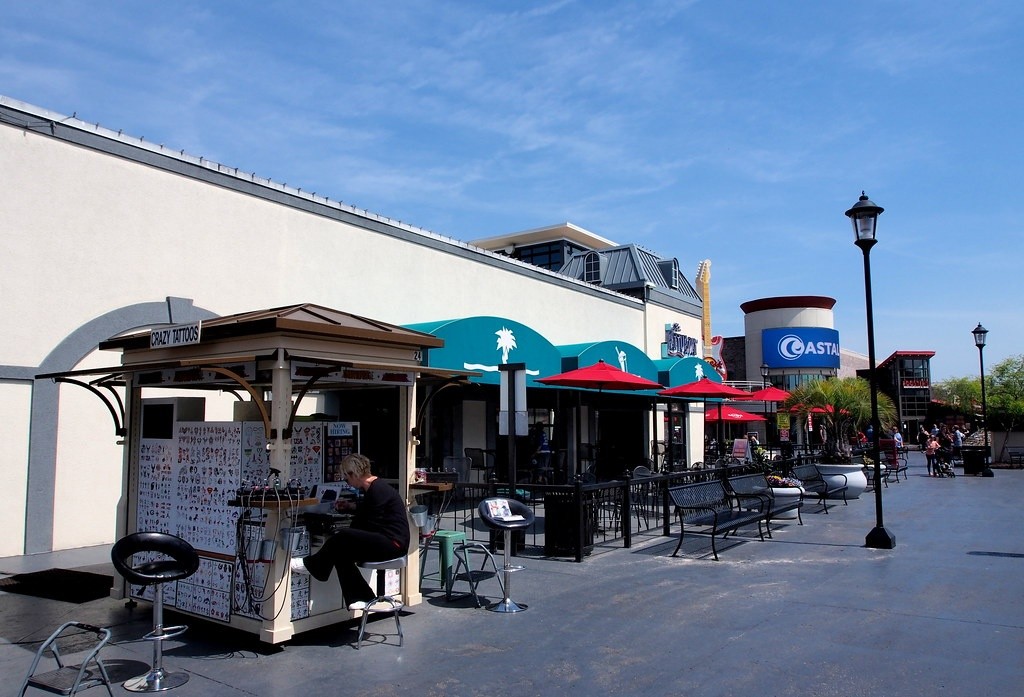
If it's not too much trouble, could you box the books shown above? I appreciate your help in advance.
[485,499,525,521]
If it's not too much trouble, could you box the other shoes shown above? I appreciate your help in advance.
[349,600,369,610]
[291,558,310,575]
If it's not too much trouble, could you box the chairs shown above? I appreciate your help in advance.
[464,444,742,545]
[15,621,115,697]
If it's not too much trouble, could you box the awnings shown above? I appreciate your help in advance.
[553,340,658,476]
[652,357,723,461]
[397,316,561,485]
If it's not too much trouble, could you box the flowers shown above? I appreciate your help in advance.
[766,475,802,488]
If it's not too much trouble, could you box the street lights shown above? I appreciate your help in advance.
[970,322,994,478]
[760,361,770,451]
[844,190,896,549]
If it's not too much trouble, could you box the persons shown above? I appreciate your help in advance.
[742,435,759,450]
[529,422,550,483]
[822,425,827,450]
[917,422,969,478]
[818,425,824,451]
[867,425,873,441]
[289,453,410,610]
[894,430,904,447]
[856,431,866,447]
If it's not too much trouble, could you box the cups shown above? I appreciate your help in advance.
[245,540,262,563]
[282,528,300,550]
[418,515,435,538]
[262,540,279,563]
[409,505,428,527]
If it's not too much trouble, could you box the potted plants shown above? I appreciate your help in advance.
[785,373,901,499]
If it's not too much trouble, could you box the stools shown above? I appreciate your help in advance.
[419,530,474,603]
[111,531,200,693]
[357,556,406,650]
[477,497,535,613]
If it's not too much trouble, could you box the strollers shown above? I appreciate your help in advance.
[934,447,955,478]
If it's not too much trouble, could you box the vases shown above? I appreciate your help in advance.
[769,488,801,518]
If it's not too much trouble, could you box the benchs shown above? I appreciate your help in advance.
[725,472,803,538]
[792,464,848,514]
[849,450,908,492]
[1009,451,1024,469]
[668,480,765,561]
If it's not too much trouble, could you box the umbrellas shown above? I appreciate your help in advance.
[532,359,664,481]
[655,374,754,469]
[729,384,791,459]
[704,406,768,445]
[776,402,851,452]
